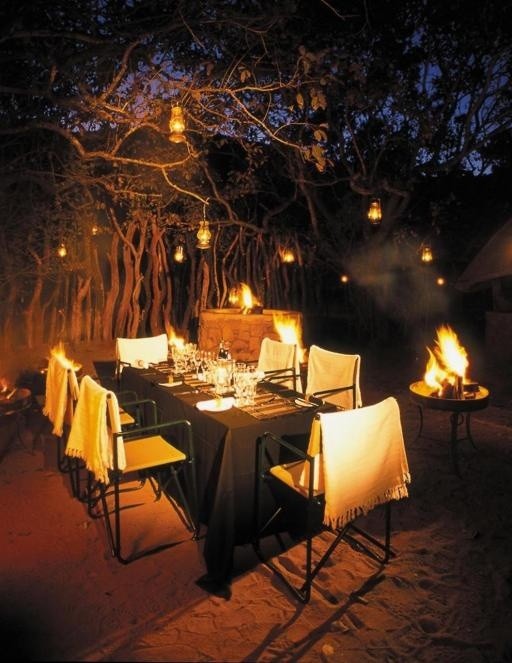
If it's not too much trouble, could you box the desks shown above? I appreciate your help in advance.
[119,357,338,580]
[1,389,33,456]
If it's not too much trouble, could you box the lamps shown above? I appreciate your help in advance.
[195,203,209,248]
[166,97,186,146]
[174,245,185,263]
[422,244,433,265]
[366,198,382,226]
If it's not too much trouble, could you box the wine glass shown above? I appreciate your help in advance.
[172,347,218,379]
[219,359,255,405]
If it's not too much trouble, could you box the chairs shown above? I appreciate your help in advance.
[63,377,204,562]
[117,334,167,369]
[278,346,362,411]
[252,396,413,603]
[42,352,143,501]
[252,336,301,393]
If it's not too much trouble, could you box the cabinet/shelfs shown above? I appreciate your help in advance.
[409,381,490,483]
